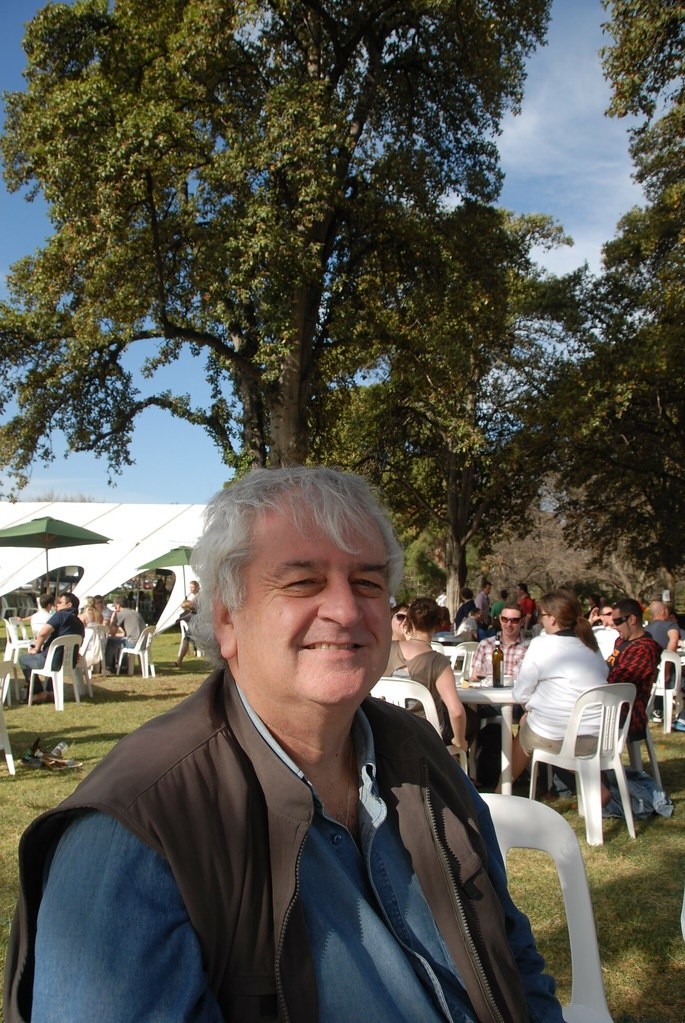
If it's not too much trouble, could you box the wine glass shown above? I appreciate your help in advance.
[475,664,486,687]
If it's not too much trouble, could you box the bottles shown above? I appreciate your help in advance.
[492,635,504,687]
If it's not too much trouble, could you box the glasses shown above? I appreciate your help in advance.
[57,602,61,604]
[601,612,612,616]
[501,617,521,624]
[613,614,630,626]
[397,614,405,621]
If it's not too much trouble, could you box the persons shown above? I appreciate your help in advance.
[377,578,685,812]
[17,592,148,708]
[127,576,171,623]
[1,464,567,1023]
[171,580,200,668]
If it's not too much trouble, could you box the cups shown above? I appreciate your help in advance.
[504,675,513,687]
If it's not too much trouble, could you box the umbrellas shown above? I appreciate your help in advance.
[0,516,113,594]
[137,546,198,600]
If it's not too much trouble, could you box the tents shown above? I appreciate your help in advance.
[0,501,214,637]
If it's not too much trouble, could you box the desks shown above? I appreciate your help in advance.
[431,632,465,643]
[443,645,475,656]
[454,680,527,798]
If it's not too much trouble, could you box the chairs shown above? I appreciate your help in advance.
[528,683,637,847]
[27,634,81,710]
[369,677,468,777]
[619,683,663,792]
[431,642,444,655]
[4,618,34,665]
[179,620,204,657]
[0,661,19,707]
[1,596,38,619]
[449,641,479,681]
[126,598,155,624]
[85,625,109,678]
[9,617,29,640]
[0,698,17,776]
[478,791,618,1023]
[655,649,682,731]
[116,626,157,678]
[77,656,95,698]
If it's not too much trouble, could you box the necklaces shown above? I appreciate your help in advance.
[334,739,353,833]
[410,638,433,650]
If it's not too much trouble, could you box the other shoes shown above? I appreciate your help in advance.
[671,718,685,730]
[168,662,179,667]
[20,694,50,704]
[652,709,663,722]
